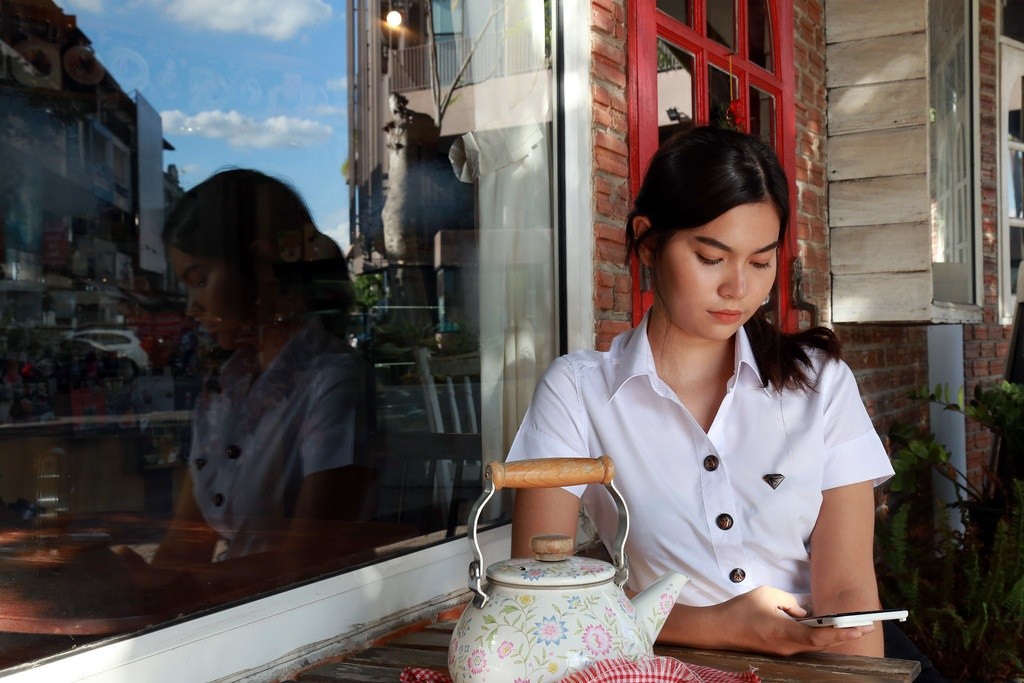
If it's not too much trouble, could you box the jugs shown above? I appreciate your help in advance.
[447,455,688,683]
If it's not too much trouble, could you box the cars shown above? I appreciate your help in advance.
[60,335,127,393]
[72,330,150,385]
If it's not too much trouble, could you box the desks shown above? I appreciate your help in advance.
[0,512,354,634]
[283,619,921,683]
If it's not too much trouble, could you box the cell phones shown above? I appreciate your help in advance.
[793,609,910,630]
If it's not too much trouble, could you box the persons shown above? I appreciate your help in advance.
[110,167,366,605]
[507,125,898,659]
